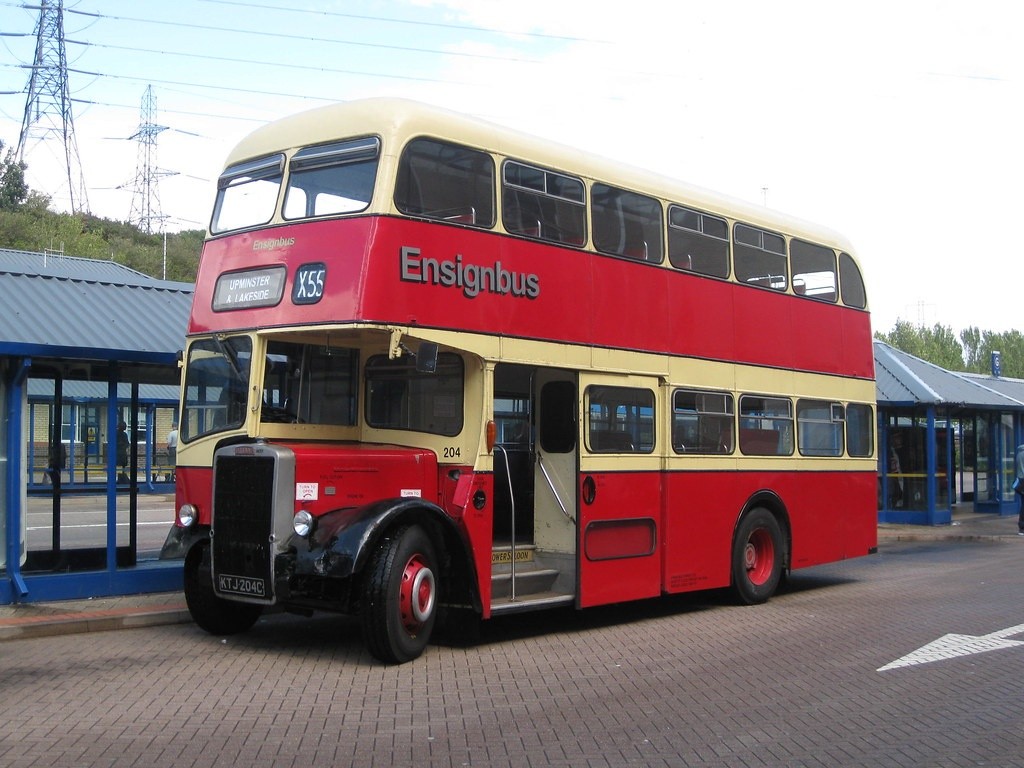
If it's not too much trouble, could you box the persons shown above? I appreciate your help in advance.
[212,355,272,428]
[43,436,67,484]
[116,422,129,466]
[1014,443,1024,535]
[167,422,178,466]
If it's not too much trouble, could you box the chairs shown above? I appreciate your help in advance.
[420,205,475,224]
[739,274,771,288]
[622,241,647,260]
[671,255,691,270]
[484,420,783,491]
[775,279,805,295]
[504,220,541,238]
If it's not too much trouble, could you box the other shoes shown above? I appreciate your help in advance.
[1017,532,1024,536]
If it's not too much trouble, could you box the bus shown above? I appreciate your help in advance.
[159,95,879,666]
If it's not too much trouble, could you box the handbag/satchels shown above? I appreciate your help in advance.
[1012,478,1024,495]
[42,473,51,486]
